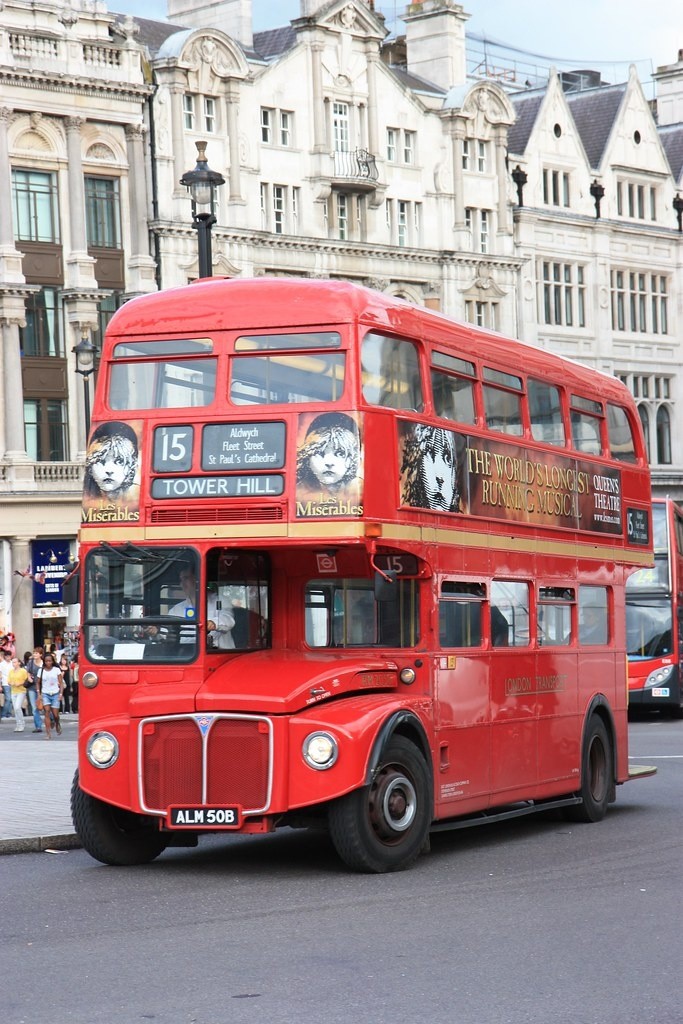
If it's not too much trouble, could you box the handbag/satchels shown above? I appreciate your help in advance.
[36,693,43,710]
[24,678,34,688]
[62,678,67,689]
[0,690,5,706]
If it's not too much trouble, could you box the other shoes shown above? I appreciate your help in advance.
[33,729,36,733]
[51,721,55,729]
[37,728,42,732]
[45,737,51,739]
[57,728,62,735]
[17,727,24,732]
[59,712,62,715]
[1,717,10,721]
[14,728,18,731]
[72,709,77,713]
[9,717,16,721]
[65,712,69,714]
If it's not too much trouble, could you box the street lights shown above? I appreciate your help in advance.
[179,140,225,281]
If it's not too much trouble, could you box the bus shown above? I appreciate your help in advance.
[62,273,652,874]
[537,496,683,717]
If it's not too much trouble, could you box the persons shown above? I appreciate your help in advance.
[0,634,79,740]
[142,566,237,649]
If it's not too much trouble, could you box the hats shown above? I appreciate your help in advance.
[305,412,361,440]
[90,422,137,452]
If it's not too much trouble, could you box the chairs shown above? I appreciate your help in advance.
[231,608,269,648]
